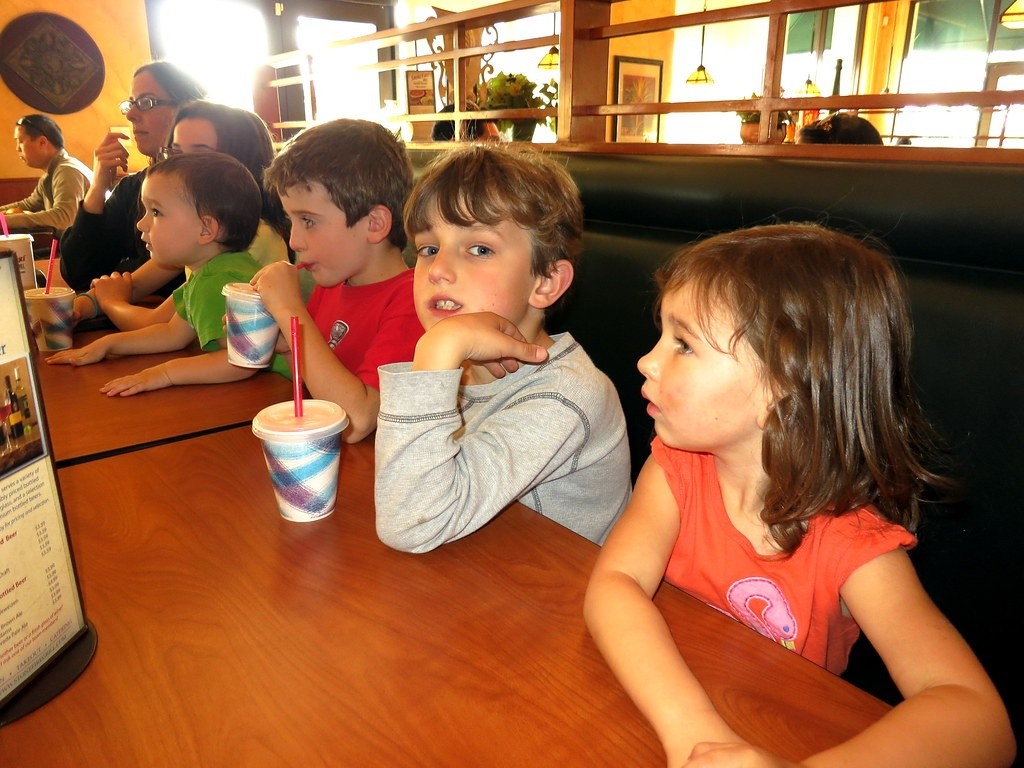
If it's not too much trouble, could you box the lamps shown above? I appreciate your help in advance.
[686,7,714,87]
[538,12,560,70]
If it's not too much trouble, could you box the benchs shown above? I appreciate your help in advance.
[400,146,1024,768]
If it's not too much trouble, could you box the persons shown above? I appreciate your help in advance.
[371,142,632,557]
[42,151,292,399]
[249,116,425,444]
[795,112,883,145]
[580,222,1017,768]
[58,62,316,306]
[430,100,502,142]
[0,114,110,233]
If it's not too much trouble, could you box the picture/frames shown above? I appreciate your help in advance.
[610,53,664,147]
[406,70,437,114]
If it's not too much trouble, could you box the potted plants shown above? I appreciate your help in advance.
[472,71,558,144]
[735,88,797,143]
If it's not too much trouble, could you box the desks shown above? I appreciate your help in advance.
[0,421,897,767]
[19,251,312,463]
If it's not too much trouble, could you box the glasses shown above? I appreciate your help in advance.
[160,146,184,159]
[118,96,175,115]
[16,116,49,141]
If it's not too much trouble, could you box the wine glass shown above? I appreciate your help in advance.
[0,389,19,455]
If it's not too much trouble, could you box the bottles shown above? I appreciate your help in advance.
[13,366,33,430]
[4,376,25,438]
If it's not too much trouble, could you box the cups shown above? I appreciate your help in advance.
[23,286,77,352]
[0,233,38,291]
[220,282,279,369]
[252,398,349,523]
